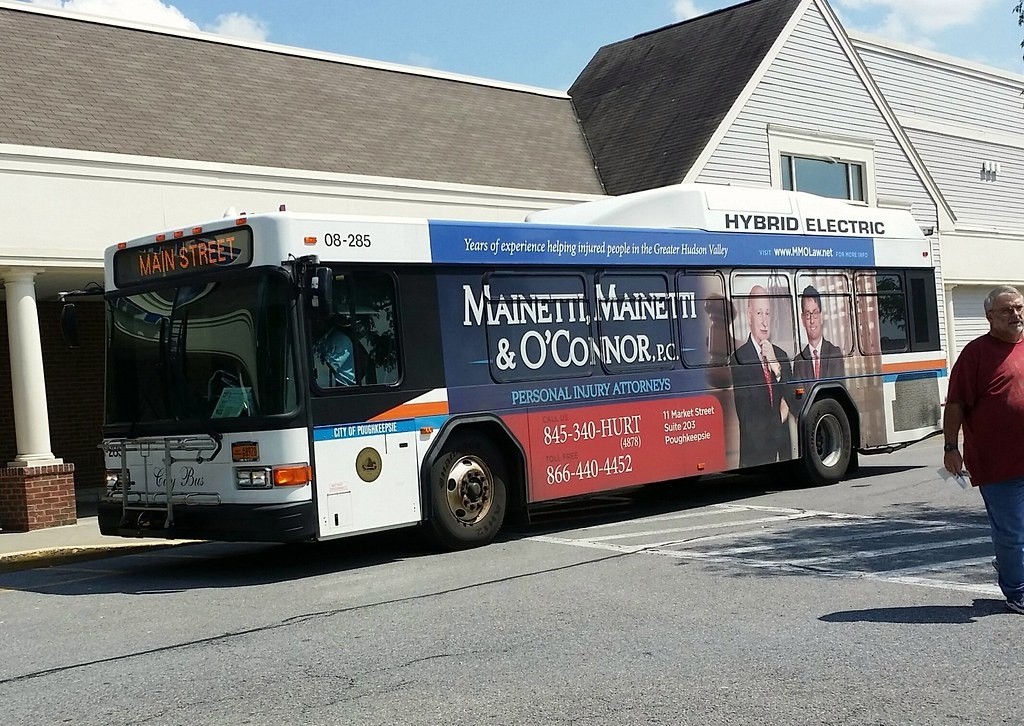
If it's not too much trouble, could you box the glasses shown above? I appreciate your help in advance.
[804,310,820,320]
[989,304,1024,316]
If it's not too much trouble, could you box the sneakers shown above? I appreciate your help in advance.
[1005,593,1024,613]
[992,558,1000,573]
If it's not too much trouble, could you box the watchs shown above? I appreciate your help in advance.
[945,443,958,451]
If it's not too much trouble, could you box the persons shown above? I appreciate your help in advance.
[729,285,846,469]
[310,296,356,387]
[945,285,1024,615]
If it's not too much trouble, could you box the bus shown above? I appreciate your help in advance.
[97,184,945,553]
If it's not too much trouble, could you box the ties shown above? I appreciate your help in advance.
[813,350,819,379]
[762,356,773,407]
